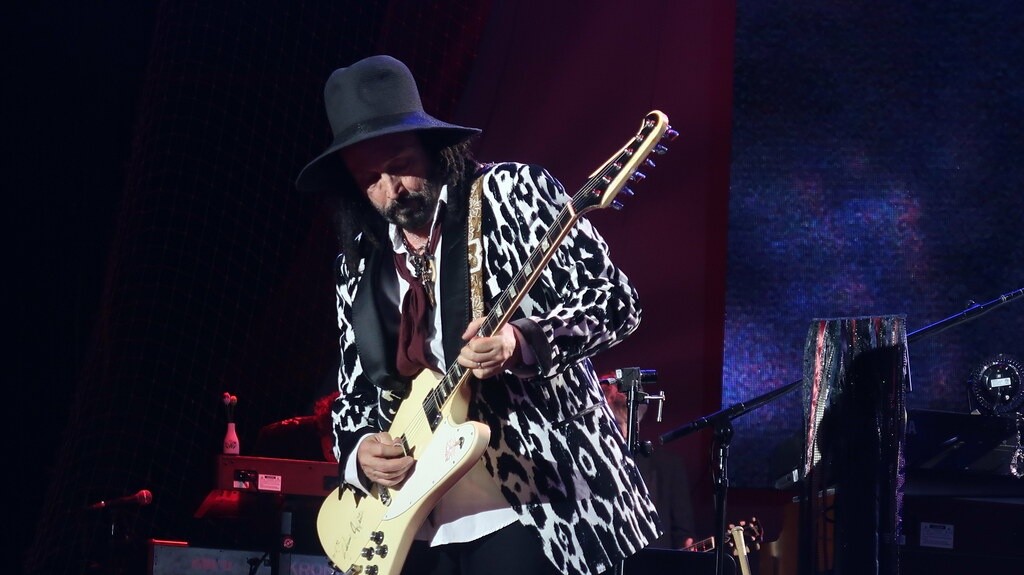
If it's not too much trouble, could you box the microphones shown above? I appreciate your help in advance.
[89,489,156,510]
[639,441,654,457]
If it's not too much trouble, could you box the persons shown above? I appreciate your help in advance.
[313,391,336,462]
[296,56,665,575]
[603,372,648,438]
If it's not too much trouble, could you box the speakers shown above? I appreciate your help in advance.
[620,548,739,575]
[888,408,1024,575]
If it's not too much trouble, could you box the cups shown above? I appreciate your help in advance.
[281,511,291,535]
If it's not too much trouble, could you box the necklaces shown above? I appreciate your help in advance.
[398,229,436,312]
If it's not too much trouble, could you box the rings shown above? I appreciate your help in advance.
[477,363,482,369]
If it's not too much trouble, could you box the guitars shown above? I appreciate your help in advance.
[312,104,684,575]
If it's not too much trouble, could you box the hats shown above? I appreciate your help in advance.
[294,54,483,189]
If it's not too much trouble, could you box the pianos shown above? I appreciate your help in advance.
[214,451,339,498]
